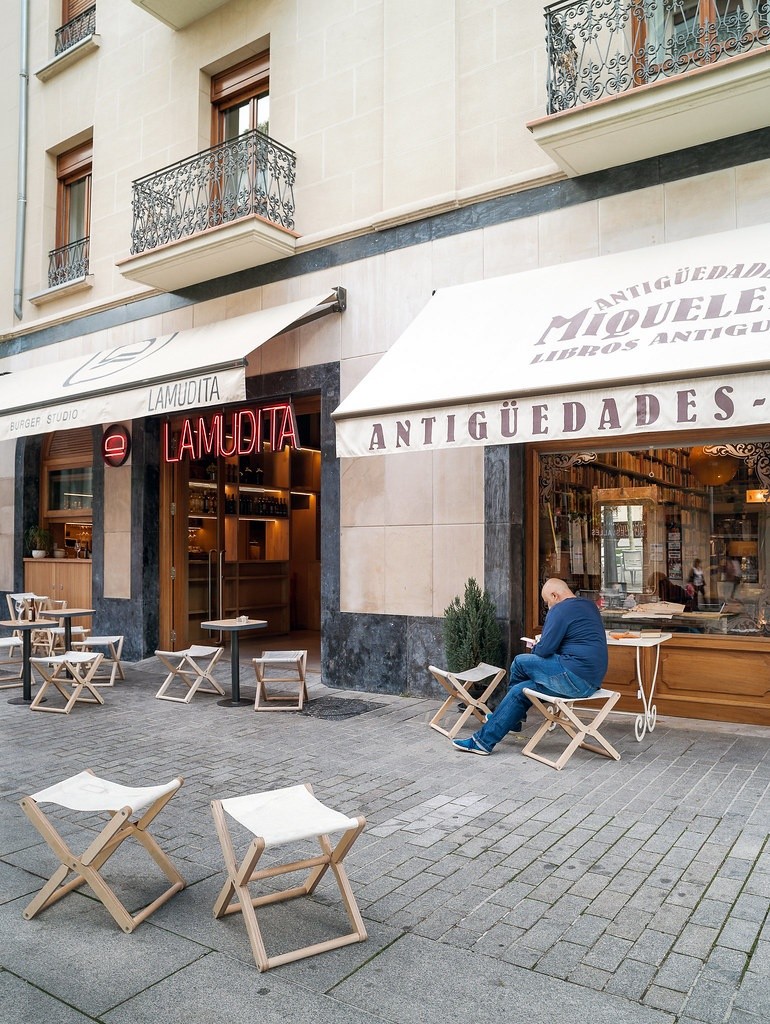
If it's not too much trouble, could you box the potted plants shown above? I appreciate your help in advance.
[26,526,50,559]
[443,576,506,715]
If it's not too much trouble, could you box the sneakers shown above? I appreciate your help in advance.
[452,738,489,756]
[485,713,522,734]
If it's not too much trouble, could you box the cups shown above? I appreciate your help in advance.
[54,549,68,557]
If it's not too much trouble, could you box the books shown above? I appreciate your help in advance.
[540,445,712,610]
[520,634,541,649]
[609,629,629,636]
[640,629,660,638]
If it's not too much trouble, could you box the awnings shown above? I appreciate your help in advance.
[332,225,770,458]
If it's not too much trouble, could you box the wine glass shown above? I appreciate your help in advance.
[76,527,92,537]
[74,543,82,559]
[15,597,26,623]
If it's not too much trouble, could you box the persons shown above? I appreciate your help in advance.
[453,577,608,754]
[0,291,333,442]
[688,559,709,609]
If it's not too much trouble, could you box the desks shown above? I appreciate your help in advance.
[201,619,268,707]
[39,608,96,678]
[0,619,58,706]
[601,609,737,634]
[537,629,674,742]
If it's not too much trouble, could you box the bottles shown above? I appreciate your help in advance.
[189,488,288,517]
[28,598,36,622]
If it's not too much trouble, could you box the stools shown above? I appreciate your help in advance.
[0,590,125,714]
[252,650,309,712]
[429,662,506,739]
[521,687,621,770]
[210,783,368,975]
[18,769,187,935]
[154,645,224,704]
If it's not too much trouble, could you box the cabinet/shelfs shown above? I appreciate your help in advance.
[22,558,92,652]
[188,419,322,647]
[539,448,770,638]
